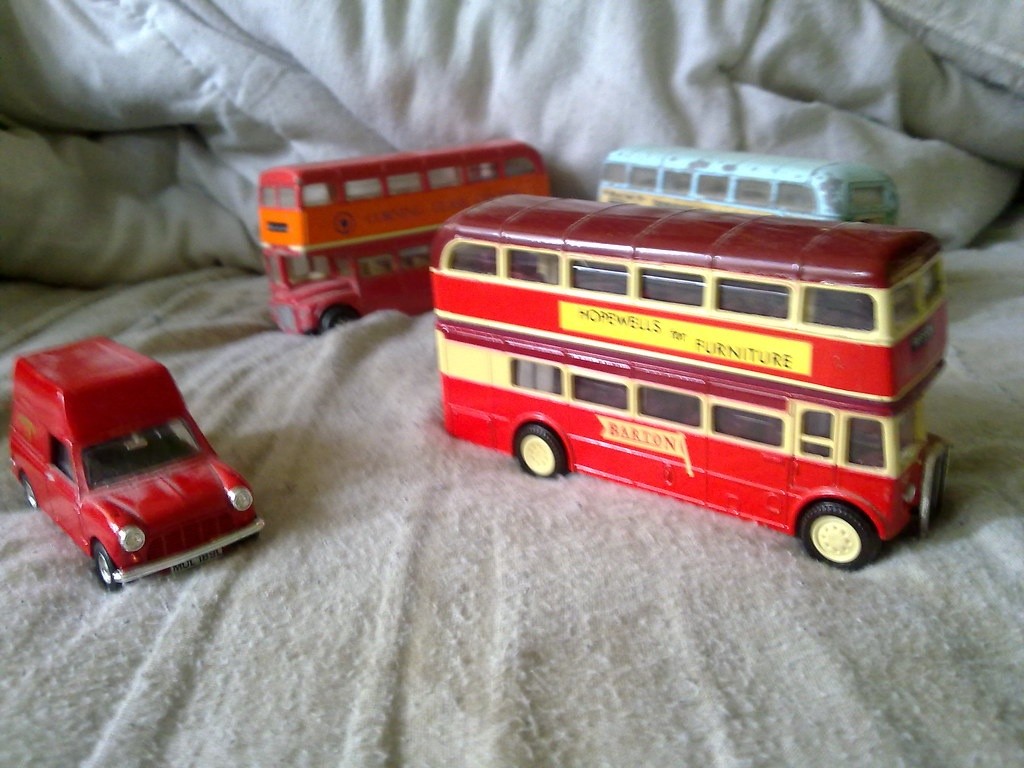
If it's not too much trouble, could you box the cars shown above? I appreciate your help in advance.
[8,334,266,593]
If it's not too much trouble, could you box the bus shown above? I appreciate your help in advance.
[430,194,956,573]
[256,137,549,338]
[597,144,901,224]
